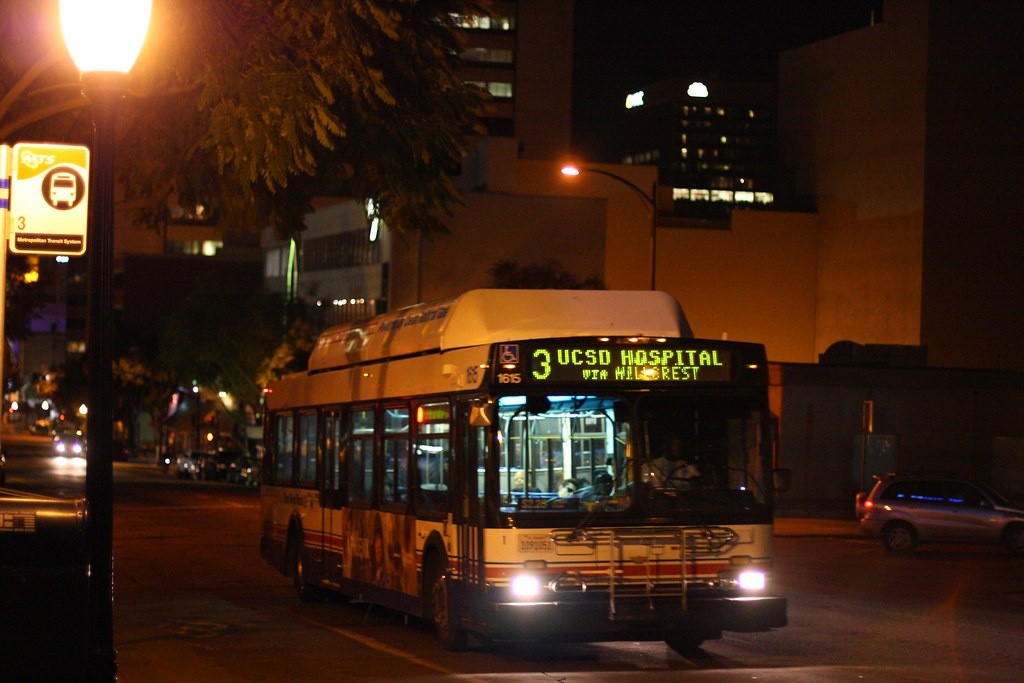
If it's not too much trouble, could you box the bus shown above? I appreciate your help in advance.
[260,286,790,651]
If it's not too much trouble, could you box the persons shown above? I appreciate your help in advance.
[594,473,613,496]
[558,479,579,497]
[511,470,540,492]
[640,433,701,488]
[373,515,386,585]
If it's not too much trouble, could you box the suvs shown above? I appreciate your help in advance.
[856,472,1024,558]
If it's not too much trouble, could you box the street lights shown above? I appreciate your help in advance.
[28,0,157,683]
[558,161,658,291]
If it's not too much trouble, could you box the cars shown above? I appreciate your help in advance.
[27,415,264,491]
